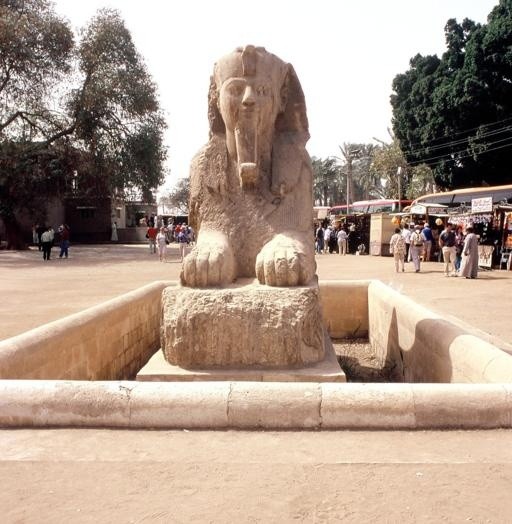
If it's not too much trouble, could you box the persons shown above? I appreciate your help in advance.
[314,223,357,256]
[32,222,70,260]
[110,221,118,242]
[146,222,195,263]
[388,221,479,279]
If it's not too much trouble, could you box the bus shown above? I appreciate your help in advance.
[312,184,512,219]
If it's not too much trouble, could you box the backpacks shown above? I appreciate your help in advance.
[413,231,425,246]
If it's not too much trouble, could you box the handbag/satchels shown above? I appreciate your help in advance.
[165,239,170,245]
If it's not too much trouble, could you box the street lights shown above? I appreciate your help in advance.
[397,167,402,213]
